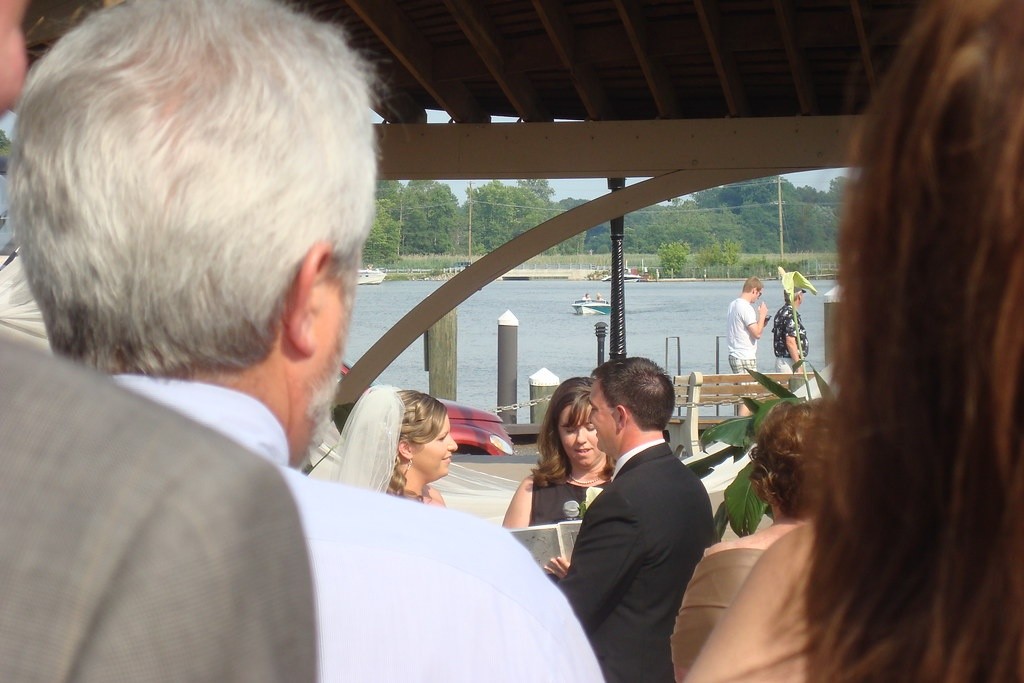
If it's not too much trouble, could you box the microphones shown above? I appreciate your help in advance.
[562,500,580,521]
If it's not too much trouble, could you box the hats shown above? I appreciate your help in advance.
[784,287,806,296]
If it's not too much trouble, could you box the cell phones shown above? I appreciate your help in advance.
[765,315,772,321]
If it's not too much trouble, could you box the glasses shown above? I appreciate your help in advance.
[758,291,762,297]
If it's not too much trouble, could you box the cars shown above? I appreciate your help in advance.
[335,360,517,460]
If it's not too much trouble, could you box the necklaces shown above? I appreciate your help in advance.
[569,473,602,485]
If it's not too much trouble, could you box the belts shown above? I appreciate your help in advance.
[778,354,801,358]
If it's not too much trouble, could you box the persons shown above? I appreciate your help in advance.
[0,2,326,681]
[675,0,1024,683]
[667,396,840,683]
[554,354,718,683]
[771,283,811,377]
[724,275,771,418]
[10,0,606,681]
[339,388,456,510]
[582,293,593,301]
[594,293,604,302]
[498,374,621,589]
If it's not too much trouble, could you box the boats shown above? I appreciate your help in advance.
[355,268,387,287]
[601,273,642,283]
[572,299,613,315]
